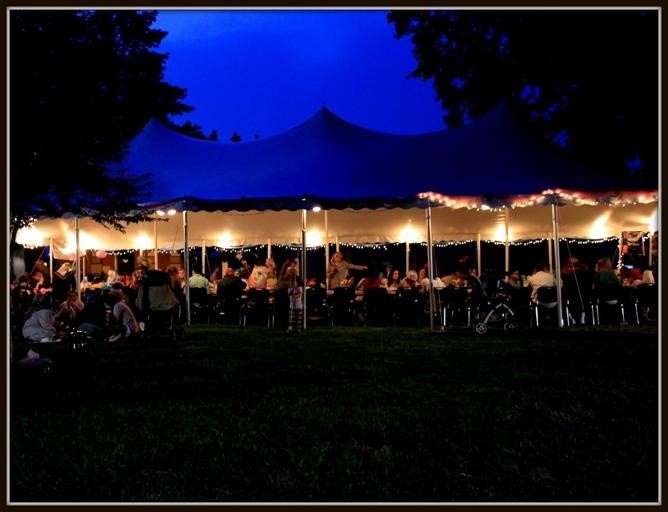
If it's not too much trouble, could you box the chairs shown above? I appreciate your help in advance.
[535,283,657,329]
[185,286,241,327]
[243,286,474,332]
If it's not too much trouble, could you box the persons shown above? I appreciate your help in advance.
[8,252,659,359]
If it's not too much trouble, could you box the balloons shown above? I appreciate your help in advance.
[96,250,106,258]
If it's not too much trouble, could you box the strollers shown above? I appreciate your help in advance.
[460,270,522,335]
